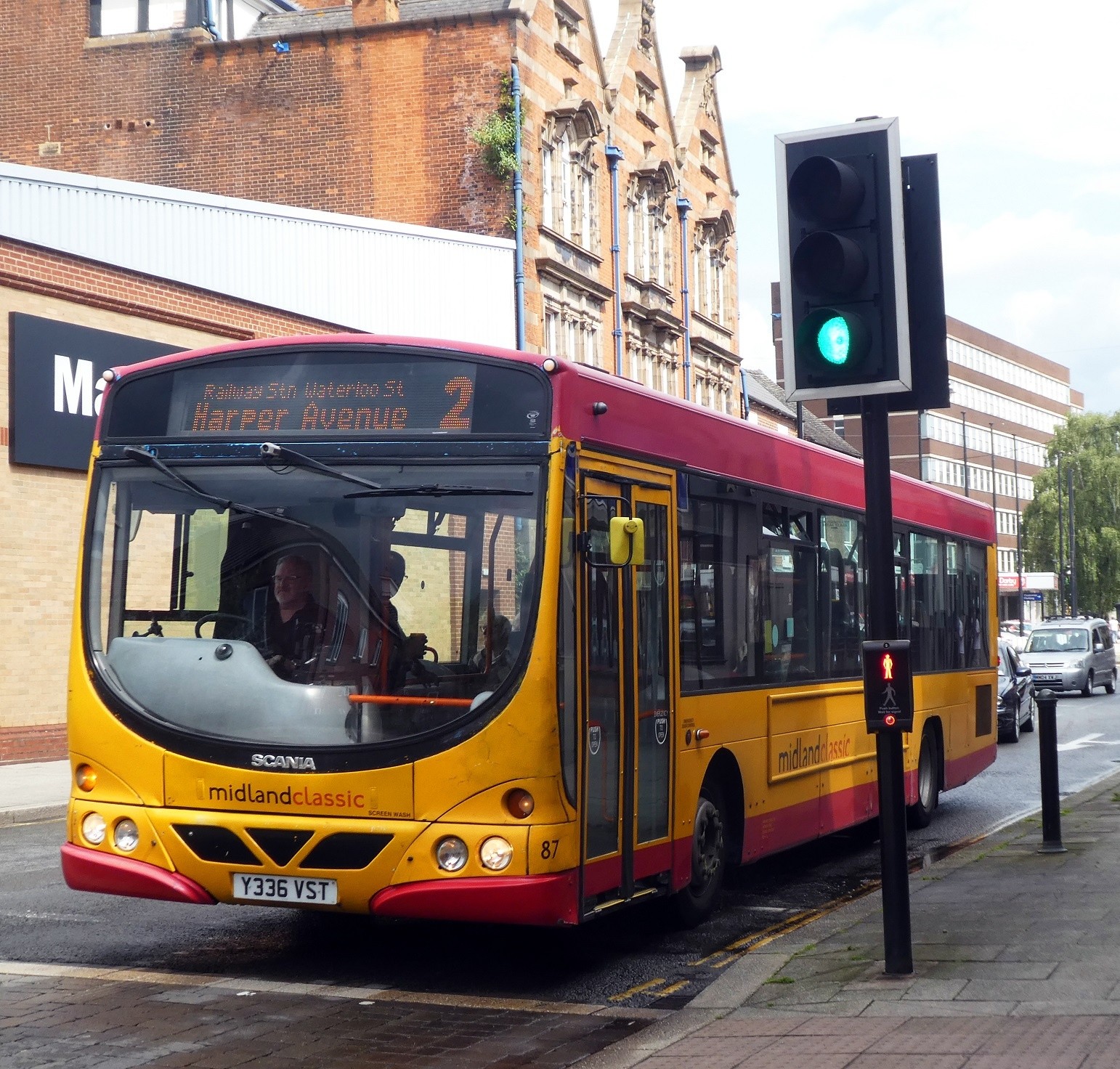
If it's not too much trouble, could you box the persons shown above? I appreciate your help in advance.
[955,610,982,668]
[241,554,357,672]
[467,615,514,680]
[384,550,428,660]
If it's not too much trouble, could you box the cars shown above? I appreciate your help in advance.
[1000,619,1031,637]
[995,638,1037,743]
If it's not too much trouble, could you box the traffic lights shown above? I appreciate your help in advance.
[860,639,914,737]
[773,120,914,401]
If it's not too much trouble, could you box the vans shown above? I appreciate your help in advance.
[1023,620,1118,698]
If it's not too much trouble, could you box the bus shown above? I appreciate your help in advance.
[59,333,1001,938]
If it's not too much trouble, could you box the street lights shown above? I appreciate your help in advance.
[1056,449,1077,617]
[959,410,1026,638]
